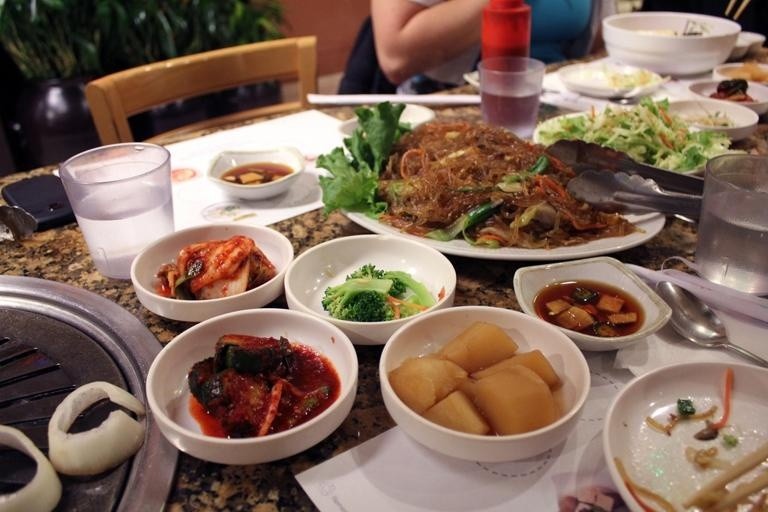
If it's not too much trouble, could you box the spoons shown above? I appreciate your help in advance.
[655,279,767,369]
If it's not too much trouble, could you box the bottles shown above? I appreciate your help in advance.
[482,0,531,77]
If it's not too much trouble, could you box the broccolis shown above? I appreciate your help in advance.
[321,263,437,323]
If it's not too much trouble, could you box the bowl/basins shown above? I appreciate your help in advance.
[600,11,743,79]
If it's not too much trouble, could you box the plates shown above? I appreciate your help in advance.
[129,224,294,321]
[207,146,300,200]
[557,63,664,99]
[513,254,672,353]
[604,360,767,512]
[283,234,458,347]
[378,305,595,463]
[143,308,360,465]
[658,27,768,145]
[537,108,700,174]
[332,196,670,268]
[336,99,438,141]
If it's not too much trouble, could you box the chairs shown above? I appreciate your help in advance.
[84,31,321,146]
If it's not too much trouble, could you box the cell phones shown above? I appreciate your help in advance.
[2,175,76,232]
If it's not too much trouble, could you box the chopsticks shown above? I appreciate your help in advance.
[680,443,766,507]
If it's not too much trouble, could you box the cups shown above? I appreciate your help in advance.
[58,143,176,279]
[697,153,768,298]
[475,54,544,140]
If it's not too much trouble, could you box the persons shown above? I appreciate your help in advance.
[337,0,616,93]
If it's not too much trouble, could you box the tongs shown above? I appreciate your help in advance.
[533,131,702,217]
